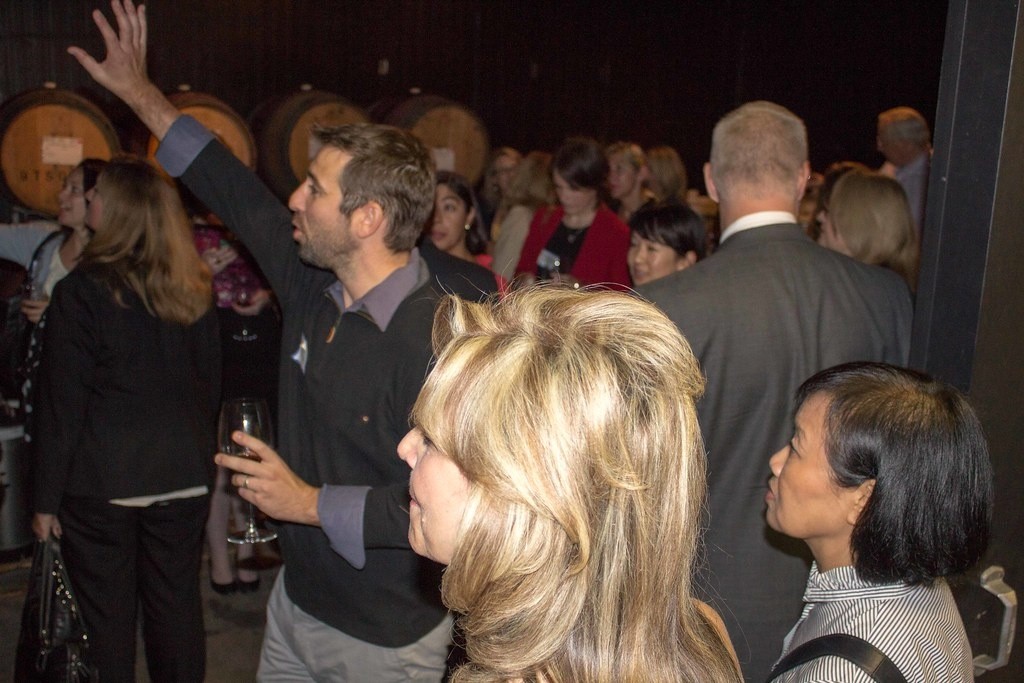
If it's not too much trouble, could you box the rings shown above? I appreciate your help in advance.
[243,476,250,488]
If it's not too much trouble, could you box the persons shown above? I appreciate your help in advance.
[398,285,744,683]
[766,362,994,683]
[0,0,937,683]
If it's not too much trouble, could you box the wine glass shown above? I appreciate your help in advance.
[231,281,258,341]
[217,395,279,545]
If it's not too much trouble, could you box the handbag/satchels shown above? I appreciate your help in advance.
[0,230,62,400]
[13,532,101,683]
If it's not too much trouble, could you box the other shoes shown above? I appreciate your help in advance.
[235,553,261,594]
[208,557,235,596]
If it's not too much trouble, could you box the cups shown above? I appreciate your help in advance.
[545,258,568,281]
[22,256,44,301]
